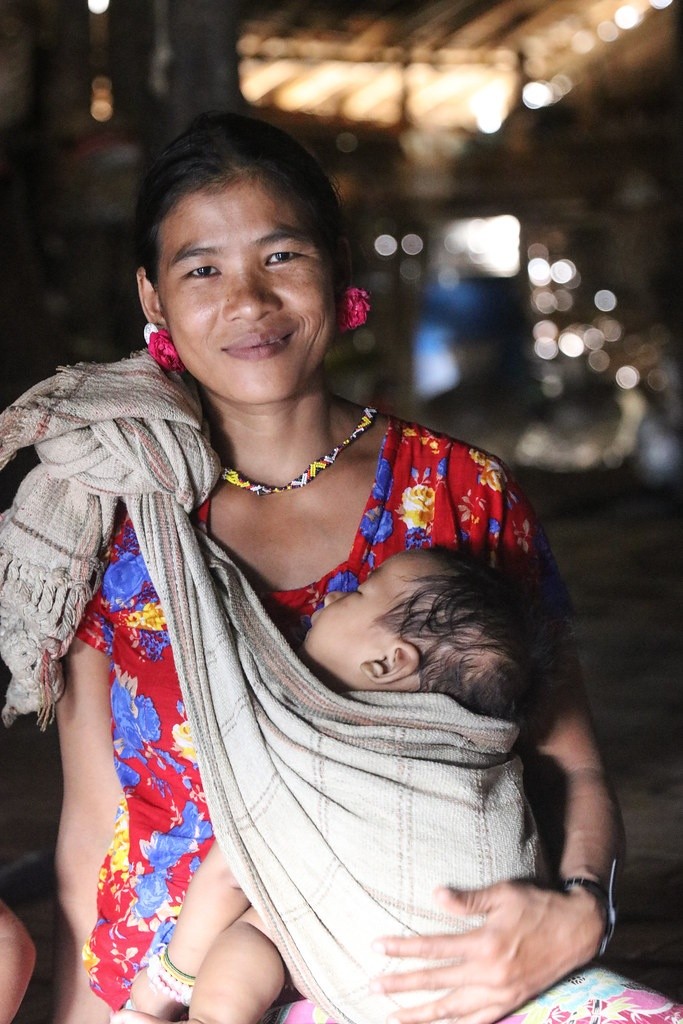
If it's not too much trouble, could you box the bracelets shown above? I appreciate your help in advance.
[146,947,194,1008]
[563,858,620,958]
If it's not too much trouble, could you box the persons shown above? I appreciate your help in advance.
[112,545,562,1024]
[0,113,683,1023]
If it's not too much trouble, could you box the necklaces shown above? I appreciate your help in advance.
[223,405,379,496]
